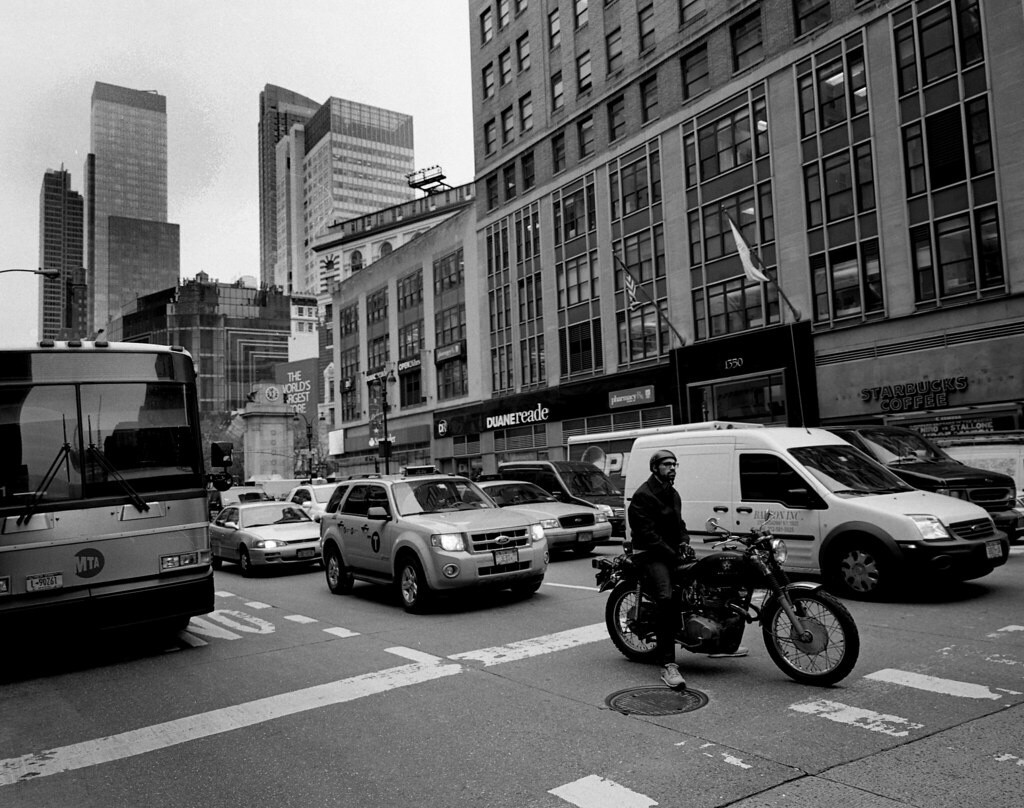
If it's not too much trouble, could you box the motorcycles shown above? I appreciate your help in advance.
[589,528,861,681]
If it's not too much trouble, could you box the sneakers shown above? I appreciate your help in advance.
[708,647,750,657]
[661,663,686,689]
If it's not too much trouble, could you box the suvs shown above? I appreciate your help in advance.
[826,426,1022,545]
[624,429,1009,601]
[317,466,550,613]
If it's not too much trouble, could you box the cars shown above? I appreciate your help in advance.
[210,499,325,577]
[289,483,386,537]
[215,475,328,513]
[433,480,613,558]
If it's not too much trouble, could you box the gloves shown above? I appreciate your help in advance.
[674,542,695,563]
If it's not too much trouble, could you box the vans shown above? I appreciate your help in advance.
[497,461,629,540]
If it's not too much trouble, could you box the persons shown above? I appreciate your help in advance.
[628,449,749,689]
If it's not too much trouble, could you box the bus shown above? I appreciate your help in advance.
[0,337,235,634]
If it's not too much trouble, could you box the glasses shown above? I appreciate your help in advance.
[660,461,679,469]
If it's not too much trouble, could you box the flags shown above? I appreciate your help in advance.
[622,267,637,304]
[728,218,770,284]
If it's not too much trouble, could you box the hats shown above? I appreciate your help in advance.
[650,450,677,473]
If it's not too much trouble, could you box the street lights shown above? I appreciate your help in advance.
[293,410,325,476]
[369,369,392,474]
